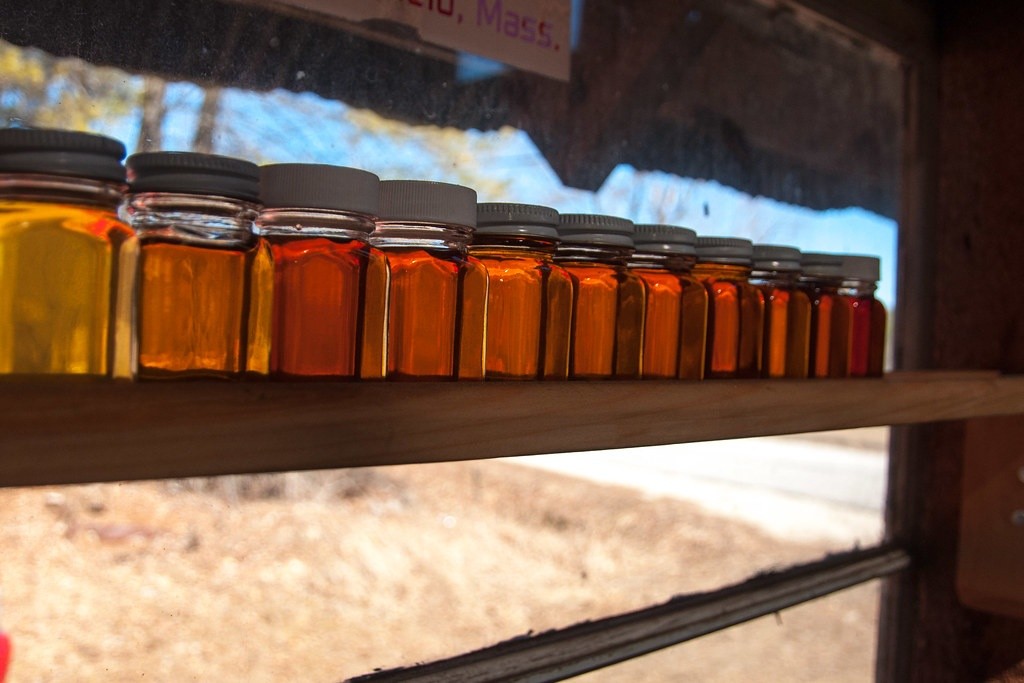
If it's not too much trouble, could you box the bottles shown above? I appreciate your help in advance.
[1,128,886,380]
